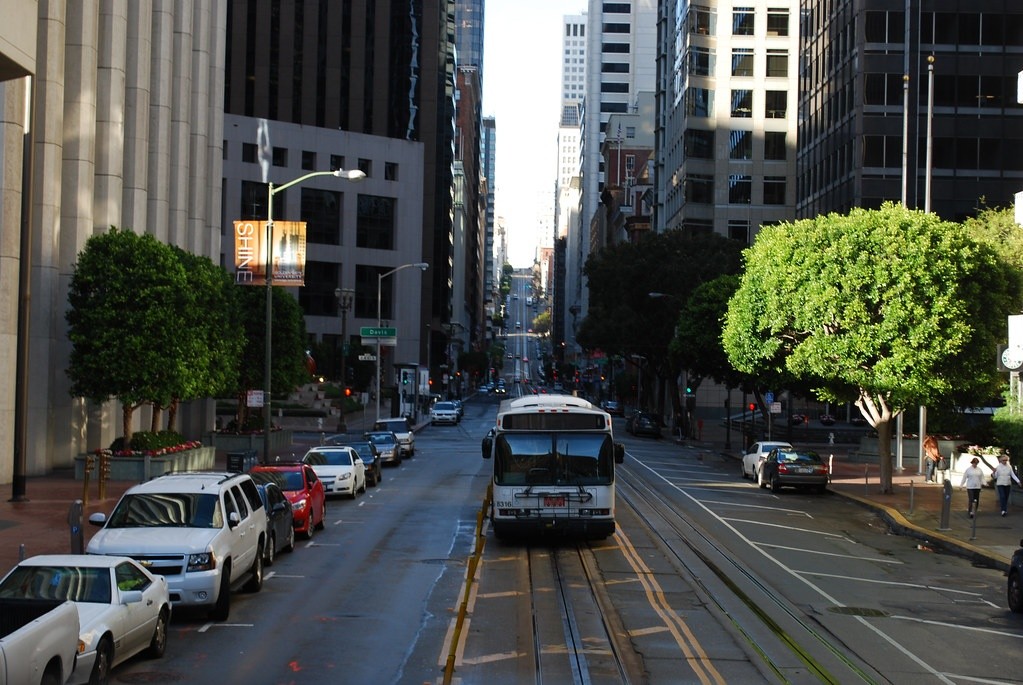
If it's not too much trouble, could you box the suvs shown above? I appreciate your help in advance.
[83,468,267,622]
[370,417,416,459]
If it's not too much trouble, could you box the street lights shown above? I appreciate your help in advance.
[263,168,367,466]
[376,263,430,420]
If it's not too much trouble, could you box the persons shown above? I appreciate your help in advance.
[991,454,1022,518]
[922,428,948,484]
[697,416,704,439]
[676,411,686,441]
[957,458,989,517]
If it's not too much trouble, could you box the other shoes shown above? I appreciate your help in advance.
[926,479,935,484]
[1002,511,1007,516]
[970,512,974,518]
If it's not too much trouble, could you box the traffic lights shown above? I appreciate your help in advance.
[344,385,354,398]
[686,383,693,395]
[749,402,757,411]
[402,370,408,385]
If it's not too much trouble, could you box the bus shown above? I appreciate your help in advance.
[482,393,626,542]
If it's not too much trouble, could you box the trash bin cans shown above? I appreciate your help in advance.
[227,450,258,474]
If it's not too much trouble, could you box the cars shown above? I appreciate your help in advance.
[335,439,381,488]
[359,431,401,467]
[603,399,625,418]
[429,399,465,427]
[515,352,521,358]
[477,376,532,395]
[625,409,662,440]
[515,321,518,328]
[507,352,513,359]
[760,446,830,495]
[523,357,529,362]
[296,445,367,500]
[512,293,519,299]
[245,461,326,540]
[0,554,172,685]
[255,480,295,566]
[741,441,799,483]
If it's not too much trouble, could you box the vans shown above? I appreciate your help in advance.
[525,296,532,307]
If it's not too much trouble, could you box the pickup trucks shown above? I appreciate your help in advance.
[0,597,81,685]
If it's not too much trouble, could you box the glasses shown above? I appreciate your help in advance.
[1002,460,1007,461]
